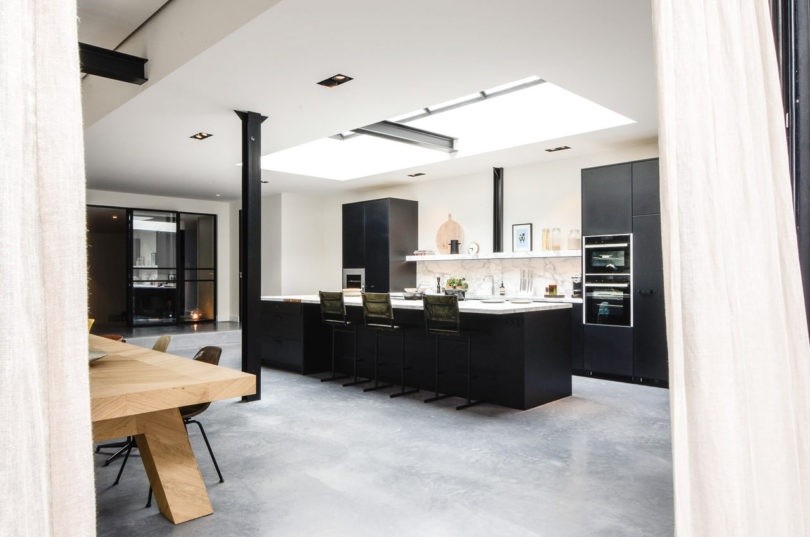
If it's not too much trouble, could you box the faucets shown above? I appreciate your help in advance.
[483,273,496,297]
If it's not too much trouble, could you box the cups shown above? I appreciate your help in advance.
[548,278,558,296]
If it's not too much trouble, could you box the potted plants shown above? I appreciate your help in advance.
[444,277,468,301]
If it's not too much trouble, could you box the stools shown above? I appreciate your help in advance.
[319,291,371,386]
[423,295,484,409]
[362,292,420,397]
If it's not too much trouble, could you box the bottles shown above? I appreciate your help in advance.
[500,281,505,295]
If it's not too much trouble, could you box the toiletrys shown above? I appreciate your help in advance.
[499,281,505,296]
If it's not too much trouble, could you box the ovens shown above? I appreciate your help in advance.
[583,231,636,328]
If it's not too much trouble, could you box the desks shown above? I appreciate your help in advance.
[89,333,256,524]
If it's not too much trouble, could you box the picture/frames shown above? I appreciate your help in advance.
[512,223,532,252]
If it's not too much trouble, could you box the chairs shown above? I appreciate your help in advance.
[97,336,172,466]
[106,346,224,507]
